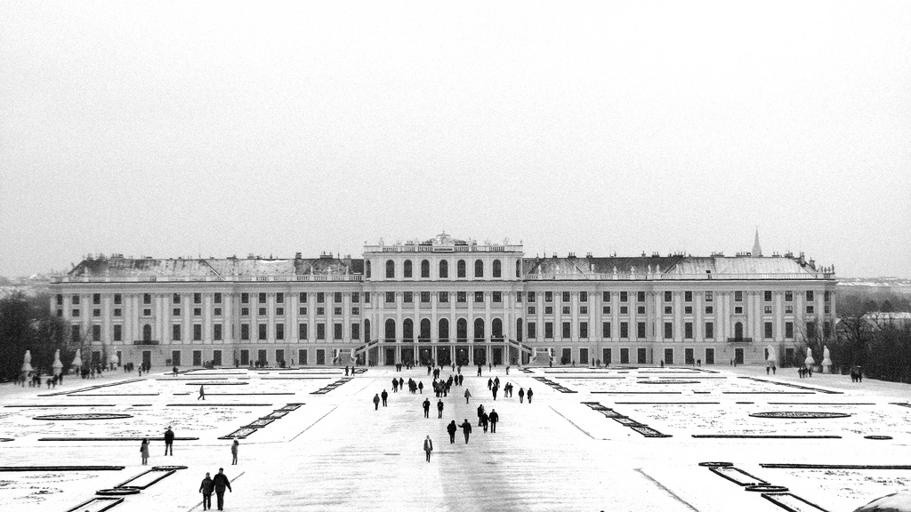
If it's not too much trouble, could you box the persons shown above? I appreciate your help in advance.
[199,473,213,511]
[730,358,737,367]
[165,426,174,456]
[231,440,239,465]
[550,361,552,367]
[345,358,533,463]
[596,358,600,369]
[202,360,215,370]
[766,361,770,376]
[697,359,701,366]
[661,360,663,368]
[197,385,205,400]
[604,357,610,368]
[850,362,864,383]
[235,358,294,368]
[772,362,776,374]
[12,358,178,390]
[213,468,232,511]
[798,365,814,378]
[141,439,150,465]
[572,360,576,367]
[691,358,694,367]
